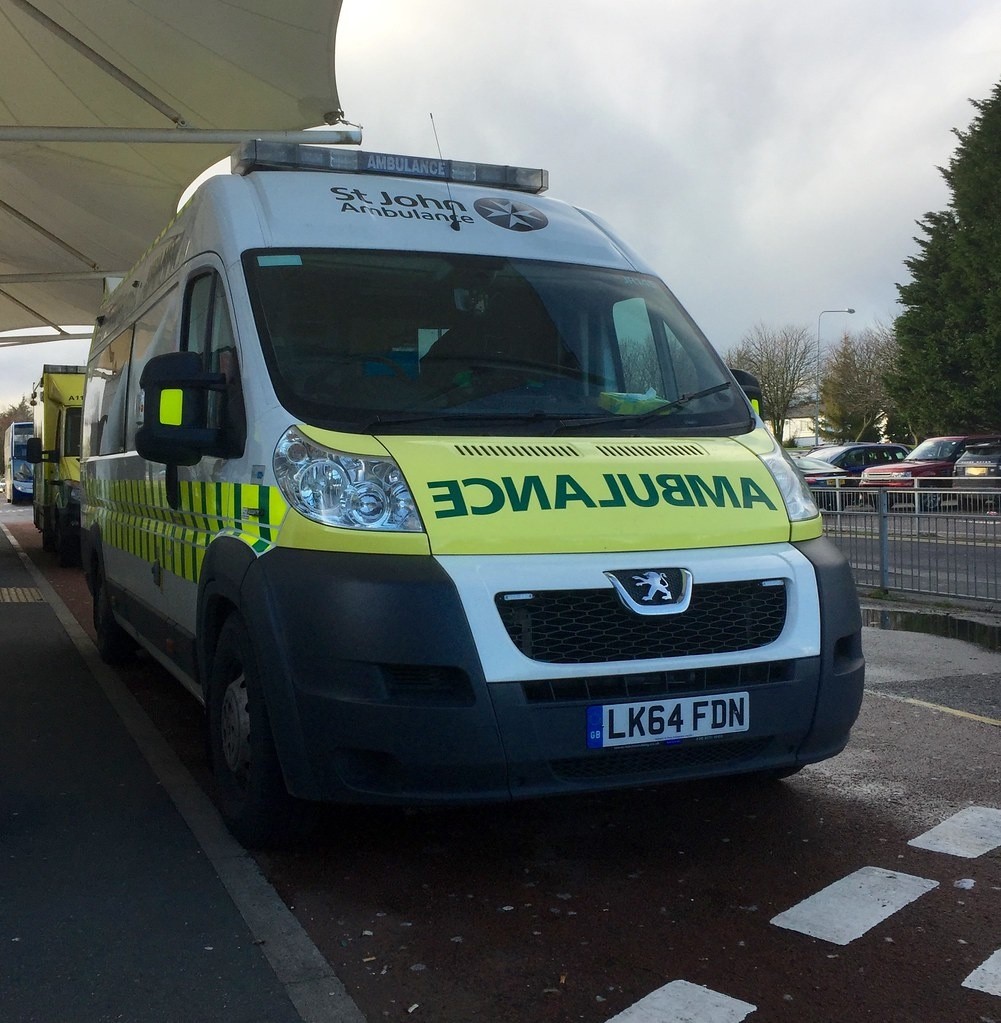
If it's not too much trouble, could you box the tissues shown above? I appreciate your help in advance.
[598,387,672,414]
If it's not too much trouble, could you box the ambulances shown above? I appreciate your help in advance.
[75,134,867,843]
[25,361,86,567]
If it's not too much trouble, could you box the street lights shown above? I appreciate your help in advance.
[815,308,855,445]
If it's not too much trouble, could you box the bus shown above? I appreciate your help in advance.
[3,422,34,504]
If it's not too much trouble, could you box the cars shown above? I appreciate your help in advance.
[787,453,858,511]
[952,441,1001,510]
[805,443,908,479]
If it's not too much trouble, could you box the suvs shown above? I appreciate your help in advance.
[858,434,1001,512]
